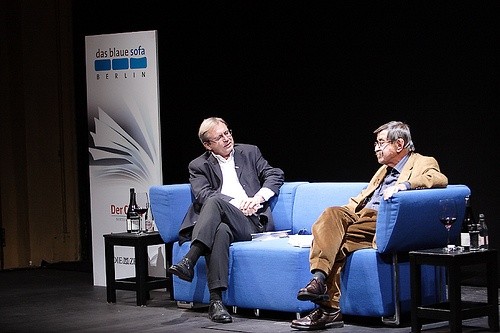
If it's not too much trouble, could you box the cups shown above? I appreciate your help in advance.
[468,224,480,250]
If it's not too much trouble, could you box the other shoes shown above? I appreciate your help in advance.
[209,299,232,321]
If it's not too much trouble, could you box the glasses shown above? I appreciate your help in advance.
[208,129,232,143]
[374,141,392,147]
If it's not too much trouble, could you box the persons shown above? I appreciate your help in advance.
[167,117,285,323]
[290,121,448,331]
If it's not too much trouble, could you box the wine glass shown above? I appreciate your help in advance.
[133,192,148,236]
[439,198,456,252]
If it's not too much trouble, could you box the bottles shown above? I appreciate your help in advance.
[478,214,488,249]
[145,202,154,233]
[460,195,471,252]
[126,188,140,233]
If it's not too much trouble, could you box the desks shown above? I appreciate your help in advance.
[103,230,173,306]
[409,245,500,333]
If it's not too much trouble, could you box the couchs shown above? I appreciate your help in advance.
[149,182,471,324]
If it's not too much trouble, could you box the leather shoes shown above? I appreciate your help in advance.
[168,256,195,282]
[297,278,330,301]
[290,308,344,328]
